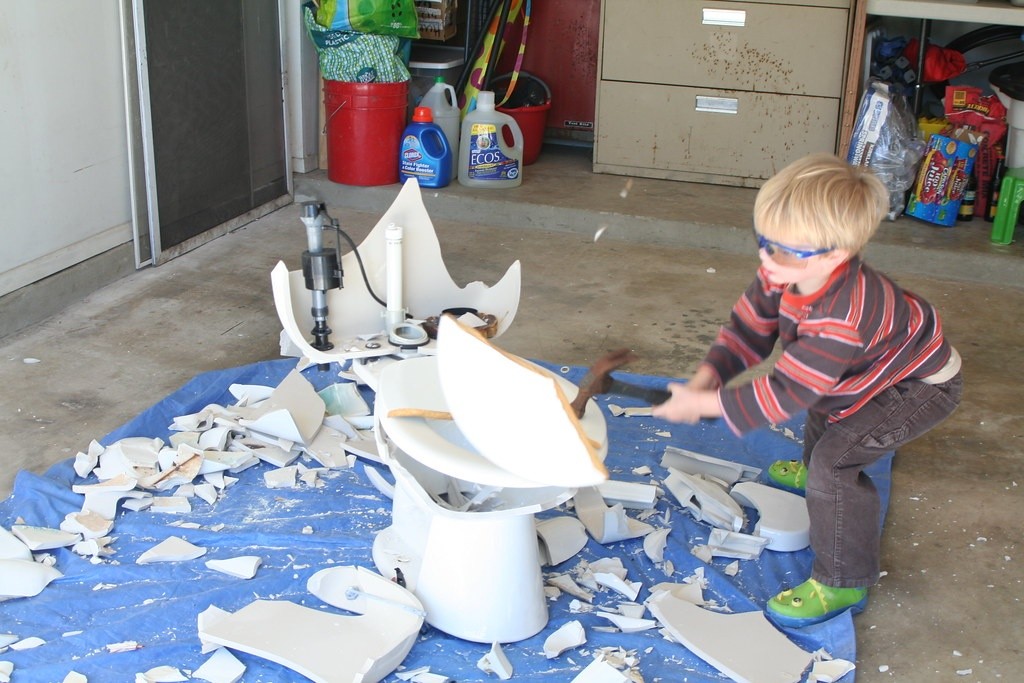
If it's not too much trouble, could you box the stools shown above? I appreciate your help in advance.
[986,165,1024,247]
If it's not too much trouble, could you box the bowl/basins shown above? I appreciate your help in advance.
[489,71,549,107]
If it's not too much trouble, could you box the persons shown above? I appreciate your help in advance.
[652,151,965,627]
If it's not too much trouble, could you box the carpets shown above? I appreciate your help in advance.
[0,358,895,683]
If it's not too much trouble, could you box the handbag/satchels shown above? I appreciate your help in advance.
[301,0,421,83]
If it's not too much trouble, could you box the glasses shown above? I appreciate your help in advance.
[751,218,833,268]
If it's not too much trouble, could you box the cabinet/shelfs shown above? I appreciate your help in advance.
[590,0,856,193]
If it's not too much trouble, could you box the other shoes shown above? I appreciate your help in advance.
[768,459,808,496]
[766,578,868,626]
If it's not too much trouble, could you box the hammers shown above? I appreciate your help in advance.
[570,346,720,422]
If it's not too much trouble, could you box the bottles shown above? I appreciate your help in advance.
[458,91,523,187]
[865,113,927,222]
[418,76,460,179]
[398,106,452,189]
[983,154,1006,222]
[957,163,978,221]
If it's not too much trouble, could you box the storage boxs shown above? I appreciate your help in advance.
[904,126,989,228]
[405,47,470,121]
[411,0,458,42]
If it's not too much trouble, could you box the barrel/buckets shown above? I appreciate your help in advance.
[321,76,411,186]
[489,101,552,166]
